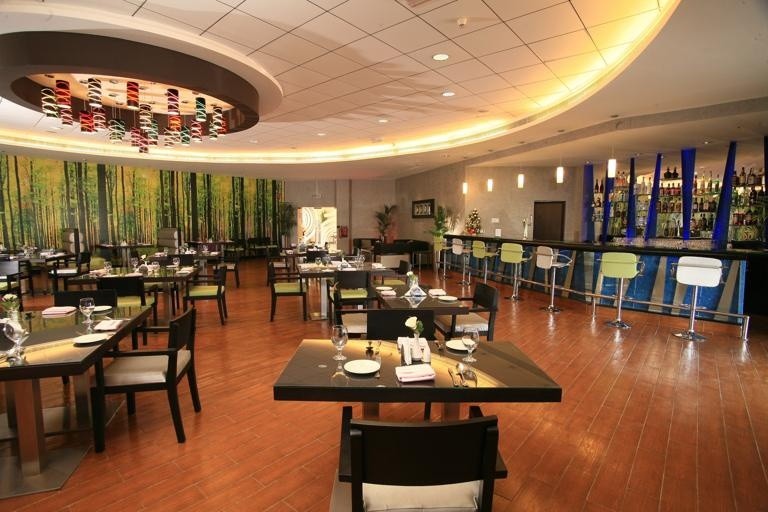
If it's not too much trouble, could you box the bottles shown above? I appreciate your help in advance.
[422,343,431,363]
[592,165,766,243]
[401,344,412,365]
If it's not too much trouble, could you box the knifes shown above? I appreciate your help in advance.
[448,367,458,386]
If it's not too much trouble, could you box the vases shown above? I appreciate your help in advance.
[467,227,477,233]
[409,335,423,362]
[4,310,26,365]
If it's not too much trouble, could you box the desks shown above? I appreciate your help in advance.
[372,281,470,340]
[274,336,563,483]
[1,302,151,501]
[298,261,394,322]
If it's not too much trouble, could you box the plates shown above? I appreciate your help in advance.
[344,359,381,374]
[444,339,475,351]
[439,295,457,301]
[375,286,392,291]
[74,333,107,343]
[93,305,112,312]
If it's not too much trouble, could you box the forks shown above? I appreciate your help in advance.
[434,340,444,351]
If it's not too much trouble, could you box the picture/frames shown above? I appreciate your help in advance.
[412,198,436,219]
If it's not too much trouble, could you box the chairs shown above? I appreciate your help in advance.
[97,276,159,346]
[325,279,367,342]
[434,283,498,341]
[244,233,433,276]
[182,264,228,325]
[368,310,434,418]
[267,261,308,321]
[348,429,500,511]
[54,291,118,353]
[372,259,410,309]
[327,269,370,327]
[93,308,202,453]
[597,250,645,333]
[340,405,507,482]
[669,255,729,345]
[2,234,241,291]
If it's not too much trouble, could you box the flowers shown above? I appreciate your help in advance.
[468,210,482,230]
[404,316,424,333]
[1,294,20,309]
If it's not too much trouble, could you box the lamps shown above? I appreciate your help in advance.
[461,112,620,195]
[38,76,228,155]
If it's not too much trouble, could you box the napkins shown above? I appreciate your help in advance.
[42,306,76,314]
[396,364,435,383]
[396,337,429,349]
[381,290,396,296]
[428,289,447,296]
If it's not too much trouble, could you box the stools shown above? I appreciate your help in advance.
[472,241,498,283]
[536,246,571,315]
[451,239,472,286]
[499,243,533,302]
[433,237,452,279]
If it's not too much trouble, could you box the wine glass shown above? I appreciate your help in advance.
[328,360,348,386]
[462,327,481,363]
[79,298,96,322]
[330,324,348,361]
[163,243,209,255]
[296,242,365,271]
[5,311,29,366]
[104,258,180,276]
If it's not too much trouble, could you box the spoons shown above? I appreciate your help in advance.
[456,363,467,386]
[365,340,374,350]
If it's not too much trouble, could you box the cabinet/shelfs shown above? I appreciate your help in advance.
[596,182,767,246]
